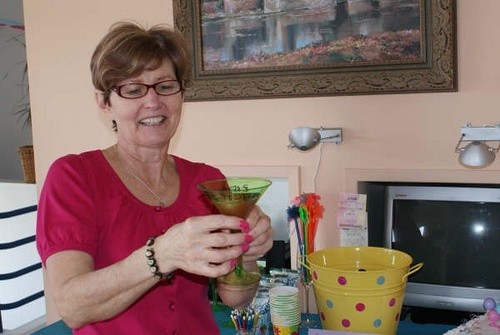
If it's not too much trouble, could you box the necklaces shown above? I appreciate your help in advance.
[115,141,171,206]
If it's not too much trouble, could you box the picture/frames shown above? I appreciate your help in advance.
[171,0,458,102]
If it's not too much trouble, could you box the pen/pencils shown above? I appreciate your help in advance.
[231,306,259,335]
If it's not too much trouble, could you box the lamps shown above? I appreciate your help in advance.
[453,123,500,167]
[287,125,342,149]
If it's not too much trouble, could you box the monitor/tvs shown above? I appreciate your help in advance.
[380,185,500,316]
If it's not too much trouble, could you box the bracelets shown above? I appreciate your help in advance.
[145,232,176,281]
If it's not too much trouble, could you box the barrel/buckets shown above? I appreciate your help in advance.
[298,245,423,335]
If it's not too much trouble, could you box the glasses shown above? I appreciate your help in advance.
[111,80,182,99]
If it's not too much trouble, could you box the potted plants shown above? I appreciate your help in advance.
[2,32,37,184]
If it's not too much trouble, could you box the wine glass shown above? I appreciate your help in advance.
[296,246,317,325]
[195,177,272,286]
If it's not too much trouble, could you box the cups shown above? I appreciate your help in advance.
[269,285,302,335]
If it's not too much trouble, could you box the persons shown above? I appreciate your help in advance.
[34,22,275,335]
